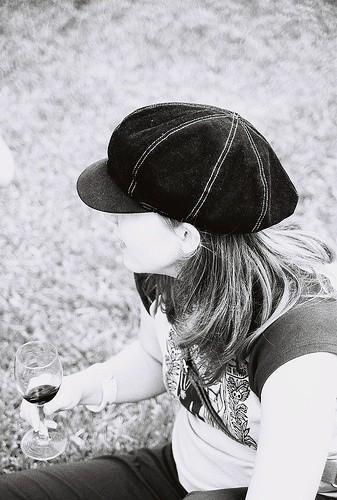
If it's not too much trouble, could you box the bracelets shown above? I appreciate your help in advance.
[83,363,117,413]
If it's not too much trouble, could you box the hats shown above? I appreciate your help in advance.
[75,102,299,236]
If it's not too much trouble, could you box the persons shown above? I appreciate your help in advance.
[0,99,337,500]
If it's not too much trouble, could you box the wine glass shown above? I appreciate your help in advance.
[13,340,68,460]
